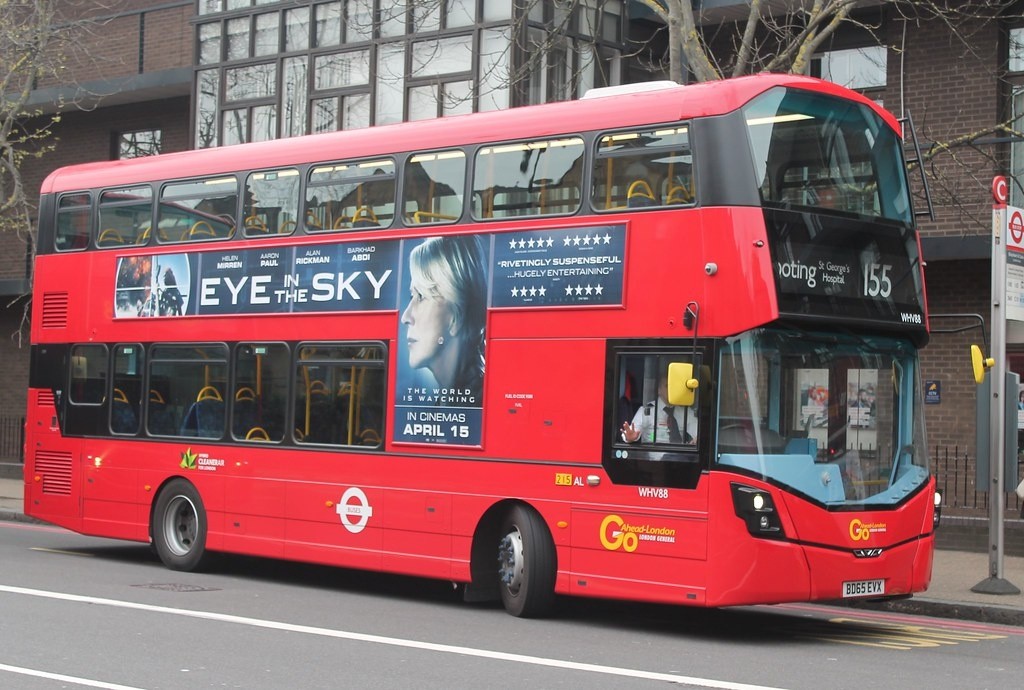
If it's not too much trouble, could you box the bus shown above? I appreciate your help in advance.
[20,69,996,619]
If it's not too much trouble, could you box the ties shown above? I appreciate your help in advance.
[662,405,684,444]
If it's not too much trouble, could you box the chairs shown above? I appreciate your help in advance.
[627,180,658,208]
[180,402,197,437]
[306,211,323,231]
[352,207,381,227]
[358,430,382,448]
[246,428,272,442]
[302,380,332,441]
[139,390,177,436]
[246,216,268,236]
[143,226,167,244]
[99,228,124,247]
[189,221,217,240]
[103,389,138,434]
[666,187,691,205]
[336,381,371,429]
[196,387,225,438]
[234,388,259,439]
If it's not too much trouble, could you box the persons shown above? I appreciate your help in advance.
[399,233,487,408]
[1017,391,1024,410]
[849,389,873,409]
[798,380,827,407]
[619,372,702,460]
